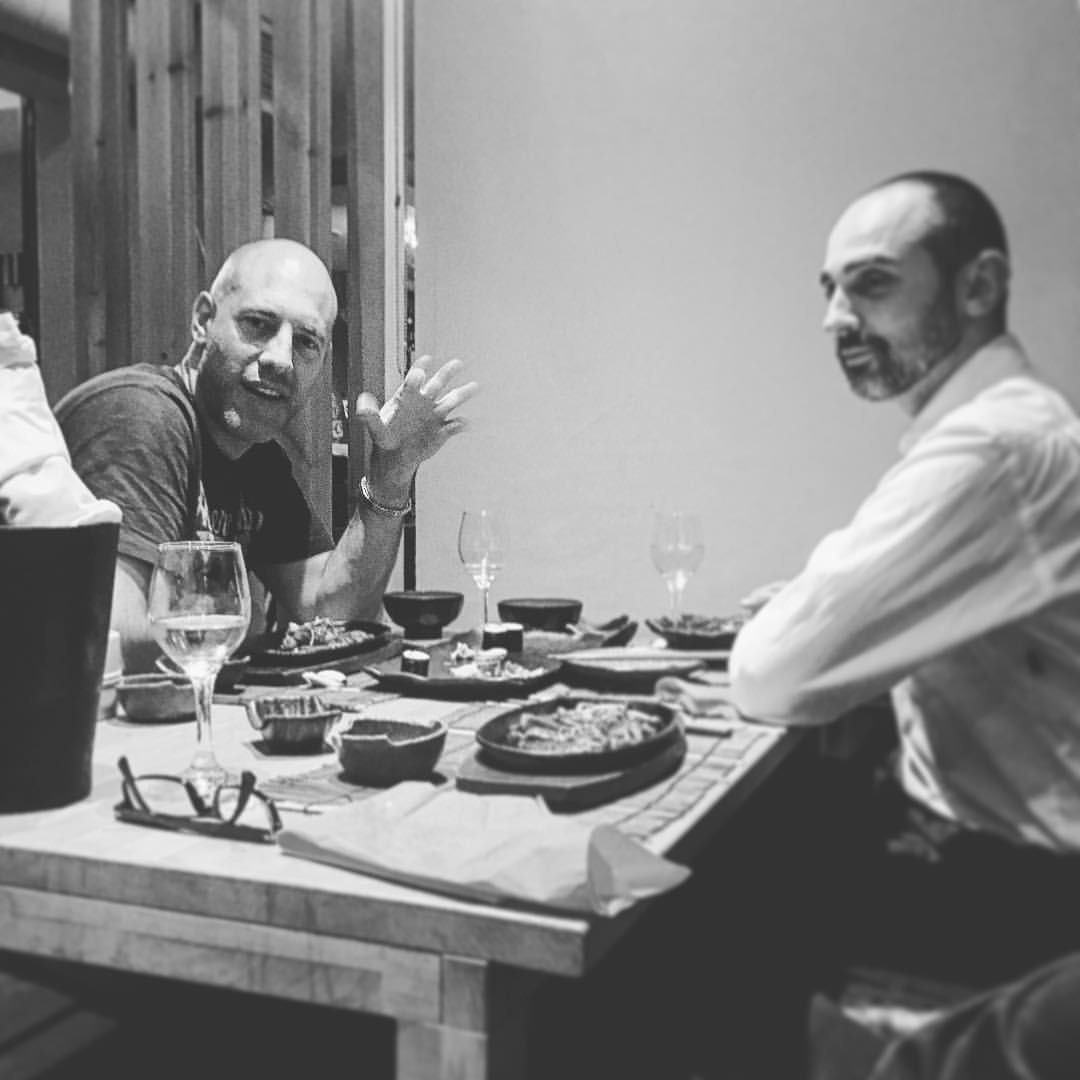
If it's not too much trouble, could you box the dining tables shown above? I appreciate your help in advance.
[0,628,817,1080]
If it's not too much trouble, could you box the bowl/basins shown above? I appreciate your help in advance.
[152,650,251,692]
[382,589,466,640]
[239,692,342,749]
[119,672,203,721]
[497,598,580,631]
[338,716,449,782]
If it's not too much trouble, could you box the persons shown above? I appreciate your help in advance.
[50,236,480,647]
[528,169,1079,1080]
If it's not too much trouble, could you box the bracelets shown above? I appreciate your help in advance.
[358,476,412,519]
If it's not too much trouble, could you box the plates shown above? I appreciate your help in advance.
[643,615,737,650]
[362,615,637,693]
[248,618,392,666]
[473,697,684,770]
[562,648,706,688]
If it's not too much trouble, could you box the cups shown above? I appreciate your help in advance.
[0,524,126,814]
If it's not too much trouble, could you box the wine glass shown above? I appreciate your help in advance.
[649,505,703,620]
[457,504,506,626]
[142,541,256,800]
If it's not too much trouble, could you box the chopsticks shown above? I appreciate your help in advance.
[545,647,732,662]
